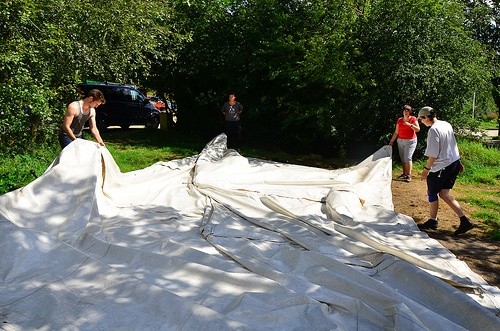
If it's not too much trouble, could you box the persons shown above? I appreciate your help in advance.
[388,104,420,182]
[416,106,474,235]
[57,87,106,150]
[221,91,245,122]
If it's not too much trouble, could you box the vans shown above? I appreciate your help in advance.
[75,83,161,130]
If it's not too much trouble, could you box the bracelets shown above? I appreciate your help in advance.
[424,166,431,171]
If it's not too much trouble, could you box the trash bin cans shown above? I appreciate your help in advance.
[159,112,173,131]
